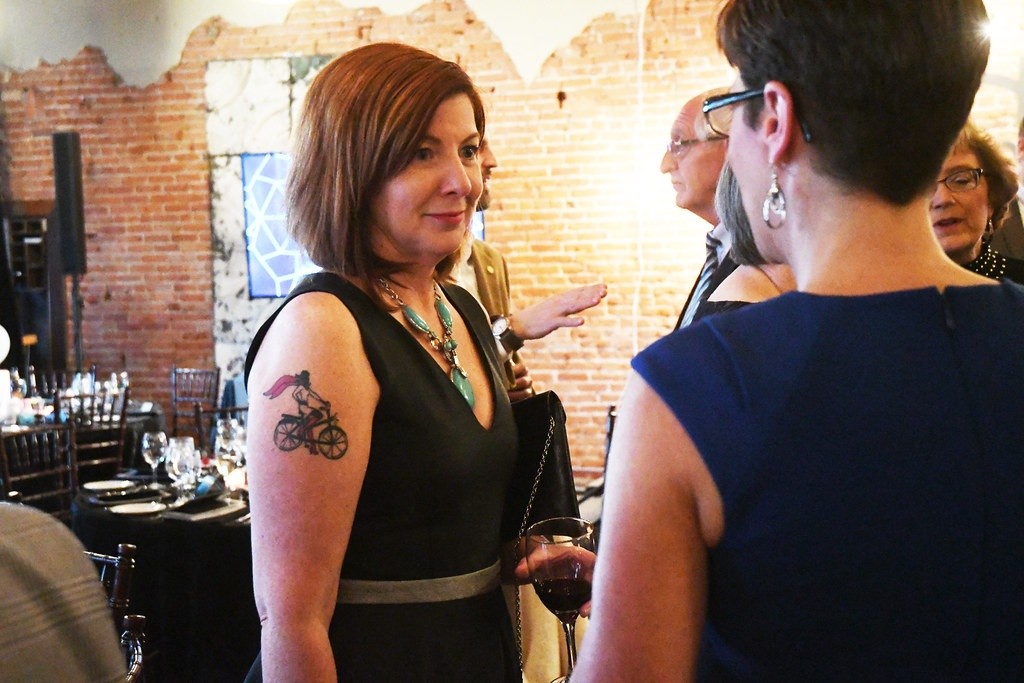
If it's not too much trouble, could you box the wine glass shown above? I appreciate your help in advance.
[524,518,598,683]
[140,430,167,489]
[215,419,246,480]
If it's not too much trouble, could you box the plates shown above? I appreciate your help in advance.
[83,480,135,491]
[109,501,167,515]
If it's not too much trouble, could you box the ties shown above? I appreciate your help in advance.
[676,229,724,330]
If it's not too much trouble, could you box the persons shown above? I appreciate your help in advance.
[243,43,596,683]
[448,133,608,403]
[928,116,1024,287]
[568,0,1024,683]
[701,157,797,316]
[661,87,736,332]
[0,503,128,683]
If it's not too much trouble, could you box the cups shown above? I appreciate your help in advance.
[165,436,201,490]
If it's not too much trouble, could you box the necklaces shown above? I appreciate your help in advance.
[379,279,475,413]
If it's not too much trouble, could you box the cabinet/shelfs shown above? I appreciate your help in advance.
[5,199,67,390]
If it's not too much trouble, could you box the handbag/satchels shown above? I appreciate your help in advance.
[498,389,583,547]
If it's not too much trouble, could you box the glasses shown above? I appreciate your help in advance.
[701,87,813,144]
[928,168,984,199]
[666,135,727,156]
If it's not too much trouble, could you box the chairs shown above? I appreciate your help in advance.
[0,364,247,683]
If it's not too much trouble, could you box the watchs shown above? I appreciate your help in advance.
[492,316,525,351]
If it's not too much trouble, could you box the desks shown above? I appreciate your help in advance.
[0,394,166,534]
[68,464,263,683]
